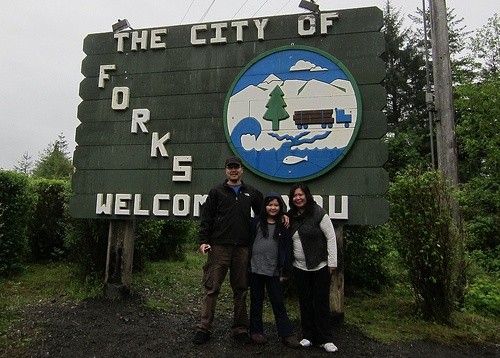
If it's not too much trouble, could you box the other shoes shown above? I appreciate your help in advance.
[282,336,300,348]
[320,343,339,352]
[233,331,251,344]
[299,339,313,346]
[250,333,265,343]
[193,332,211,345]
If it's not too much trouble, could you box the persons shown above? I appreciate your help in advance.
[288,182,340,353]
[192,155,291,346]
[246,190,301,347]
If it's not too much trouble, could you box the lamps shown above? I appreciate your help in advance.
[298,0,320,13]
[112,19,134,32]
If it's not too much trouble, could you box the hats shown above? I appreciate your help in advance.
[224,156,242,167]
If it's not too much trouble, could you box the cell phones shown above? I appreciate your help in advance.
[204,246,210,252]
